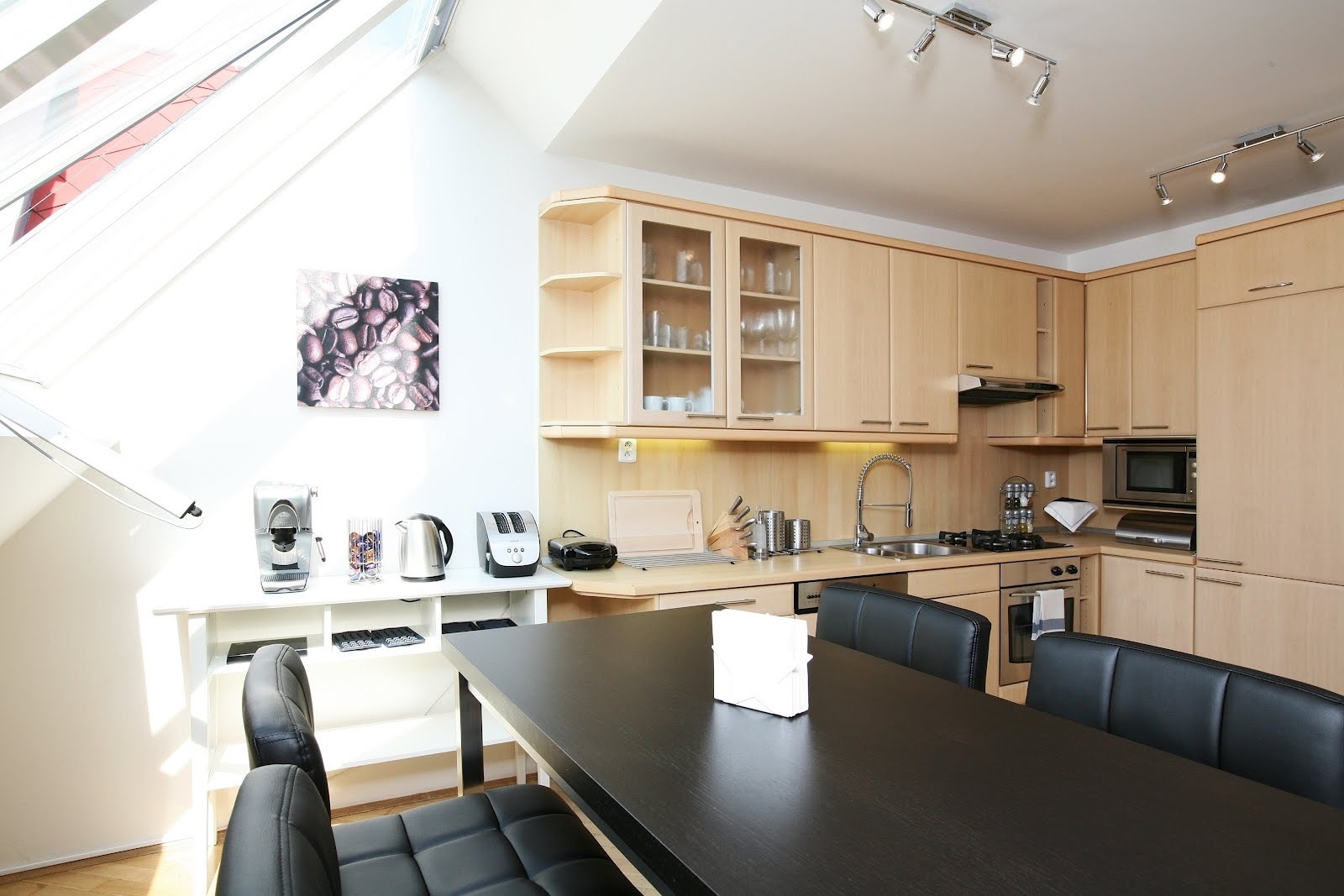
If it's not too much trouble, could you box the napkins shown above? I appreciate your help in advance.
[711,608,809,717]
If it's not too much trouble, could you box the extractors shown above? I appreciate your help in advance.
[958,374,1065,408]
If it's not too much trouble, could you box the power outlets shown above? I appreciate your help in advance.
[618,438,638,463]
[1045,471,1057,488]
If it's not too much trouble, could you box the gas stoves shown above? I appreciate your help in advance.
[923,529,1081,589]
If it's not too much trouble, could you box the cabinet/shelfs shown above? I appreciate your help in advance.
[539,185,1344,708]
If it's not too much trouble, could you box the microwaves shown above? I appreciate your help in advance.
[1101,436,1198,516]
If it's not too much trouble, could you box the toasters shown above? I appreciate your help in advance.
[476,509,541,578]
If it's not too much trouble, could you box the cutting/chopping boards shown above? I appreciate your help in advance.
[607,489,704,558]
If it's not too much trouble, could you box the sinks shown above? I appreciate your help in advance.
[828,544,906,561]
[880,540,989,555]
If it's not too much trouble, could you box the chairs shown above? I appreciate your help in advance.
[815,582,992,693]
[216,643,645,896]
[1024,631,1344,807]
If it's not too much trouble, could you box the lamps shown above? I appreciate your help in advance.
[1026,60,1052,106]
[1156,175,1173,205]
[908,15,937,65]
[1296,132,1325,163]
[991,38,1024,67]
[862,0,896,32]
[1211,155,1228,183]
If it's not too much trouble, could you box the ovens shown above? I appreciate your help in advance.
[999,579,1082,686]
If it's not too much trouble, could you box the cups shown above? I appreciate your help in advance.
[643,395,694,413]
[741,260,793,296]
[642,309,711,354]
[347,516,383,586]
[641,242,657,279]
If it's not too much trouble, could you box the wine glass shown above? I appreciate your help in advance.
[738,306,801,358]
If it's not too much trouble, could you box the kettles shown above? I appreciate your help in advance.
[395,514,454,582]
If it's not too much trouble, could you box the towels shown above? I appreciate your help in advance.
[1030,588,1066,641]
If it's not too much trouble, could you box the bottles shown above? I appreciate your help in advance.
[1001,482,1035,533]
[675,249,686,283]
[691,262,703,283]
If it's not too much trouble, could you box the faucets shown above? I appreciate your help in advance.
[853,453,914,547]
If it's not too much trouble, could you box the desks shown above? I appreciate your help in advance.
[153,547,572,896]
[440,601,1344,896]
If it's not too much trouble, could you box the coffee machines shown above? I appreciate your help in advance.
[253,480,326,593]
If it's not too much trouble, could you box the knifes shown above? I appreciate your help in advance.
[729,496,758,548]
